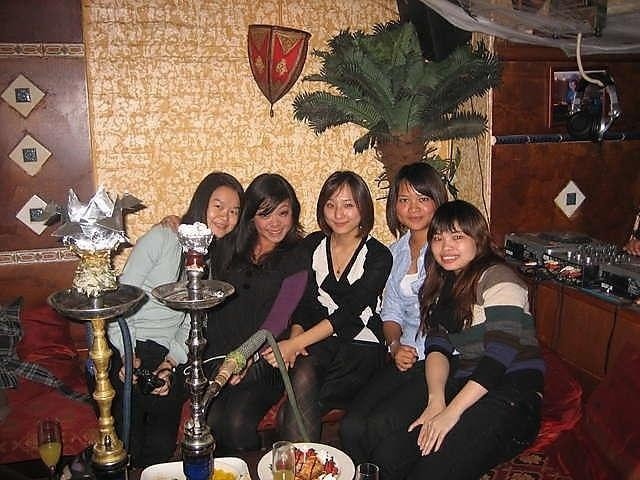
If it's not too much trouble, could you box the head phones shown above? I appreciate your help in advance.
[566,72,623,142]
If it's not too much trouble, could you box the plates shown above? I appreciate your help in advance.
[140,456,251,479]
[257,442,356,480]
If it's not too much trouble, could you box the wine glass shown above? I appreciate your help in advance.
[36,421,63,480]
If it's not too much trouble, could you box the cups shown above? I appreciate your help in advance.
[355,463,380,480]
[272,441,296,479]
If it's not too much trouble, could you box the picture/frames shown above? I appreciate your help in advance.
[548,65,612,128]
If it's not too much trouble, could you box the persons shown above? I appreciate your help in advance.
[261,170,395,443]
[68,170,245,480]
[160,172,308,454]
[370,199,547,479]
[337,163,450,461]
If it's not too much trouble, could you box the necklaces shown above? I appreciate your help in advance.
[332,235,357,276]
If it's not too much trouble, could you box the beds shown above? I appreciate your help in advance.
[0,302,102,462]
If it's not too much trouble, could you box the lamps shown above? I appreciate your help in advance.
[247,0,312,118]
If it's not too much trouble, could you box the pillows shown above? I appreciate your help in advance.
[15,302,80,363]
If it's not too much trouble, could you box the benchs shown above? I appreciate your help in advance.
[177,346,585,460]
